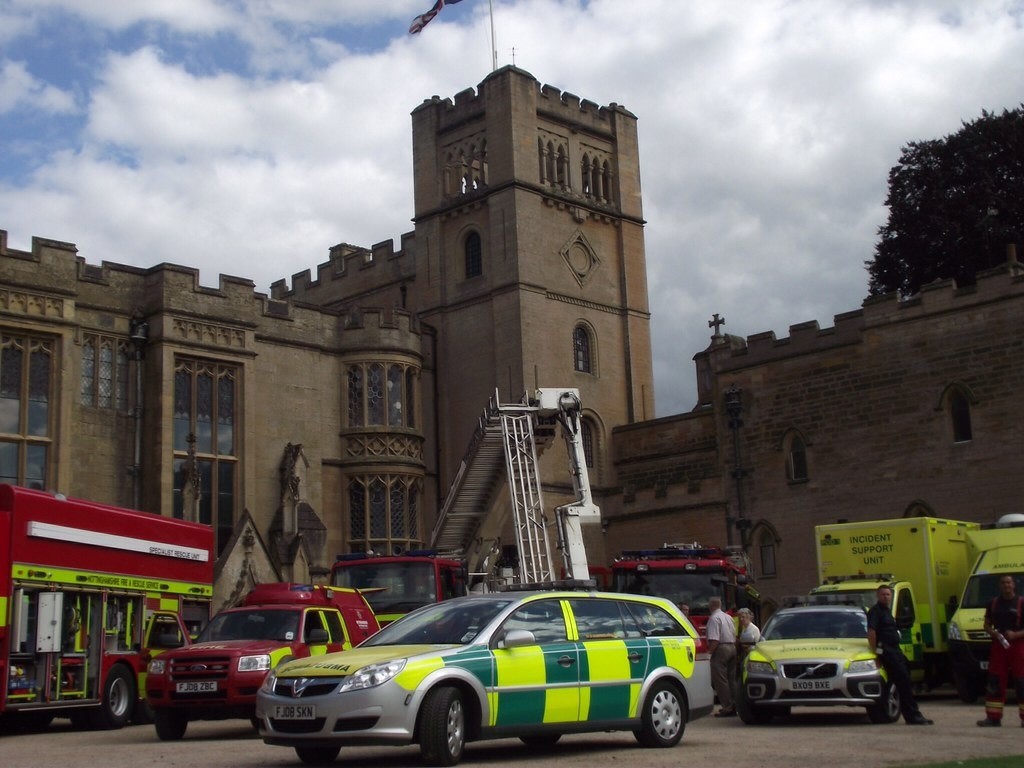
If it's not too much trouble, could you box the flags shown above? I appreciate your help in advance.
[409,0,463,34]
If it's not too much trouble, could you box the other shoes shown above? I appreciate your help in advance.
[977,717,1001,726]
[714,708,737,716]
[907,715,934,724]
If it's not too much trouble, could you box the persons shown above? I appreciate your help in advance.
[734,607,761,668]
[866,585,934,725]
[707,598,738,718]
[677,601,690,617]
[976,574,1024,728]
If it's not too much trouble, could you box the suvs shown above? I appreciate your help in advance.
[143,582,385,740]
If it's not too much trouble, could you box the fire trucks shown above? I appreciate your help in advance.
[330,387,602,630]
[560,540,762,661]
[0,482,215,733]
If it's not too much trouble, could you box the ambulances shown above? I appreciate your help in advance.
[947,524,1024,698]
[250,588,716,767]
[737,605,904,724]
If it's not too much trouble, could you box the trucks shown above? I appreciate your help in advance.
[807,516,983,656]
